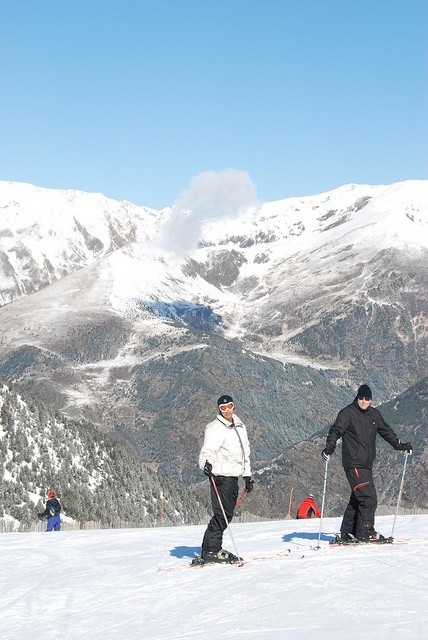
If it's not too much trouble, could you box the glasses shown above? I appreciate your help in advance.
[355,396,370,401]
[217,401,234,412]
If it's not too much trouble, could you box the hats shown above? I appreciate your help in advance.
[357,384,372,398]
[216,394,234,413]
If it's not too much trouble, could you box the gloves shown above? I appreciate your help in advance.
[320,444,335,461]
[393,441,412,456]
[202,463,212,477]
[37,513,43,518]
[243,476,254,493]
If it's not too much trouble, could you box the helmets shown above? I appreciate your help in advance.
[307,494,314,499]
[48,491,55,498]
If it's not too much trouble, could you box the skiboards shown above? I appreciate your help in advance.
[158,549,305,573]
[310,538,410,551]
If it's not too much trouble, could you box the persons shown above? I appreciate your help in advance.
[324,383,412,545]
[198,395,255,566]
[296,493,321,520]
[37,487,61,531]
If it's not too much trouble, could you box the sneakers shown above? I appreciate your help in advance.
[355,532,379,540]
[341,531,356,542]
[202,548,238,562]
